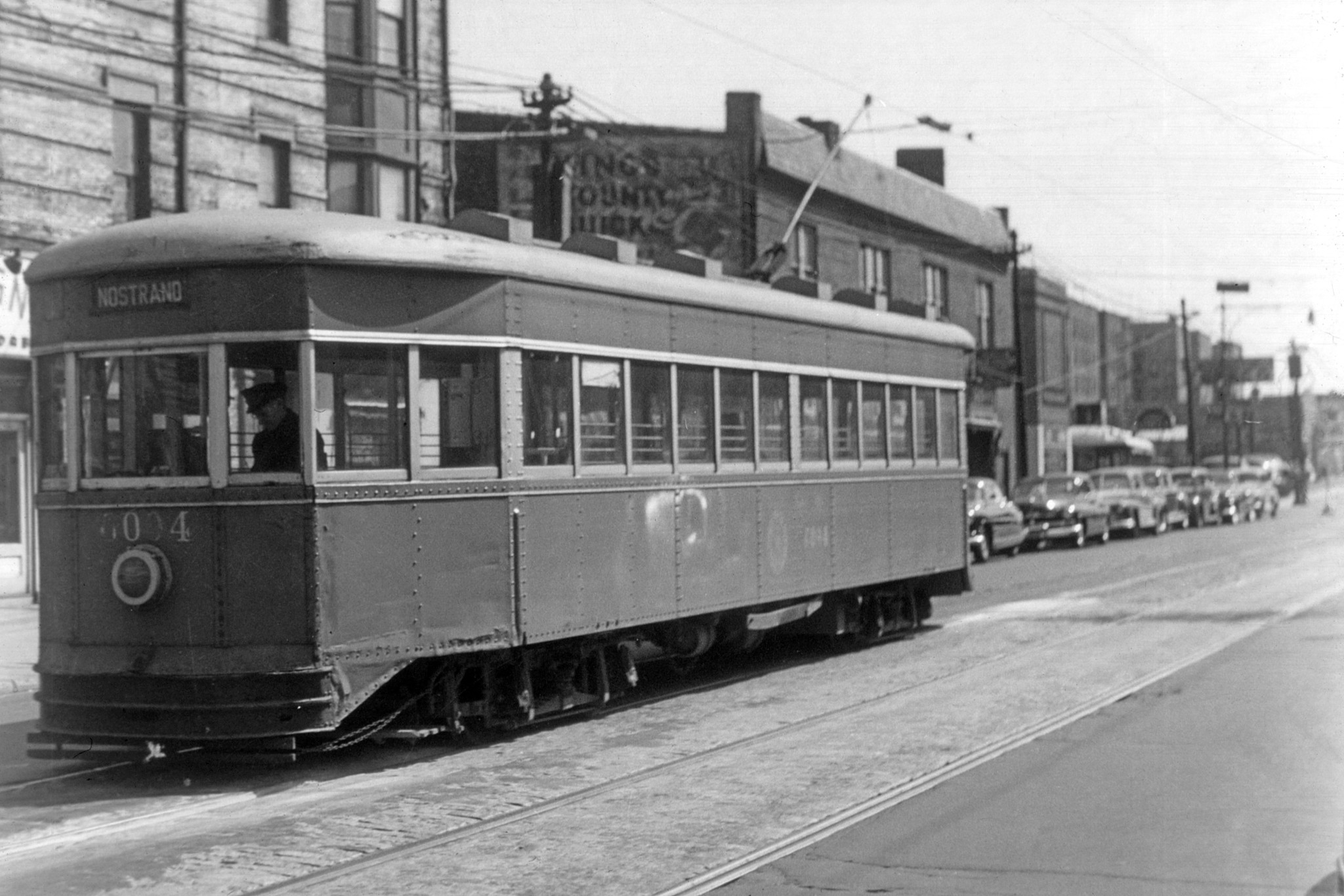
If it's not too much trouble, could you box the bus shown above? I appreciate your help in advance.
[22,97,977,759]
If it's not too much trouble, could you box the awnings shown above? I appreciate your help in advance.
[1072,426,1154,456]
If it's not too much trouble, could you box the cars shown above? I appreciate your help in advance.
[1207,454,1287,522]
[1087,468,1160,538]
[1010,472,1111,549]
[1170,465,1225,528]
[965,477,1030,563]
[1137,467,1194,532]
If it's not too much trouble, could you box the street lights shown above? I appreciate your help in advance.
[1217,281,1251,472]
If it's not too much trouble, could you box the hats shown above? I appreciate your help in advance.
[242,383,281,413]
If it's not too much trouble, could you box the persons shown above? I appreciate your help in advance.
[241,382,328,472]
